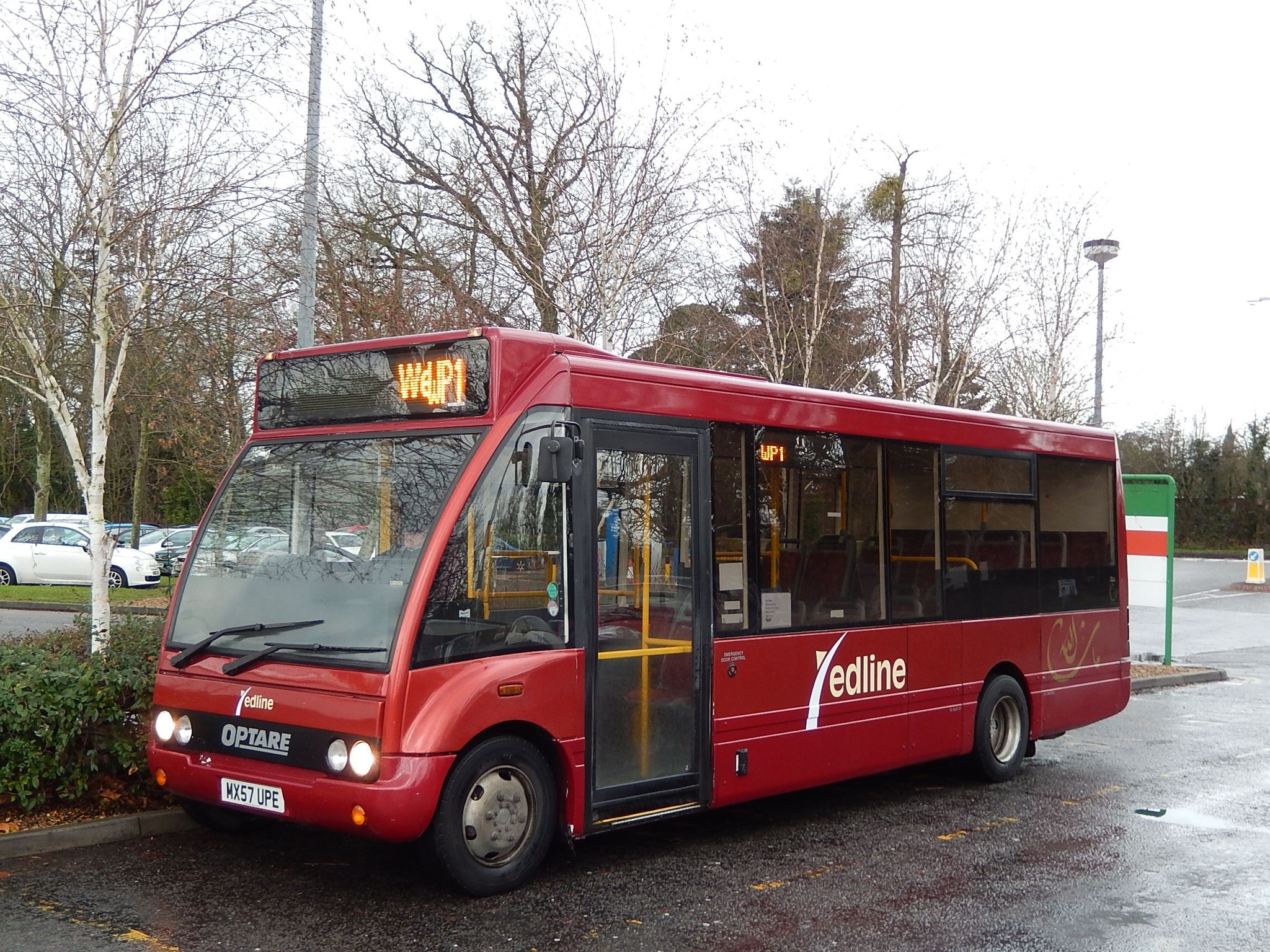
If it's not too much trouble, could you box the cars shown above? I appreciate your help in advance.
[491,535,529,571]
[334,523,370,536]
[0,522,160,588]
[307,530,376,563]
[0,513,290,578]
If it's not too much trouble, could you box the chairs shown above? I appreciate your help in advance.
[756,533,925,628]
[604,532,1067,711]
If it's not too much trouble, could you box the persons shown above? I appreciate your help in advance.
[367,520,427,582]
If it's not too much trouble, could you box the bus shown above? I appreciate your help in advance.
[148,325,1131,902]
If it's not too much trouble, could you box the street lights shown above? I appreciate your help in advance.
[1082,238,1119,427]
